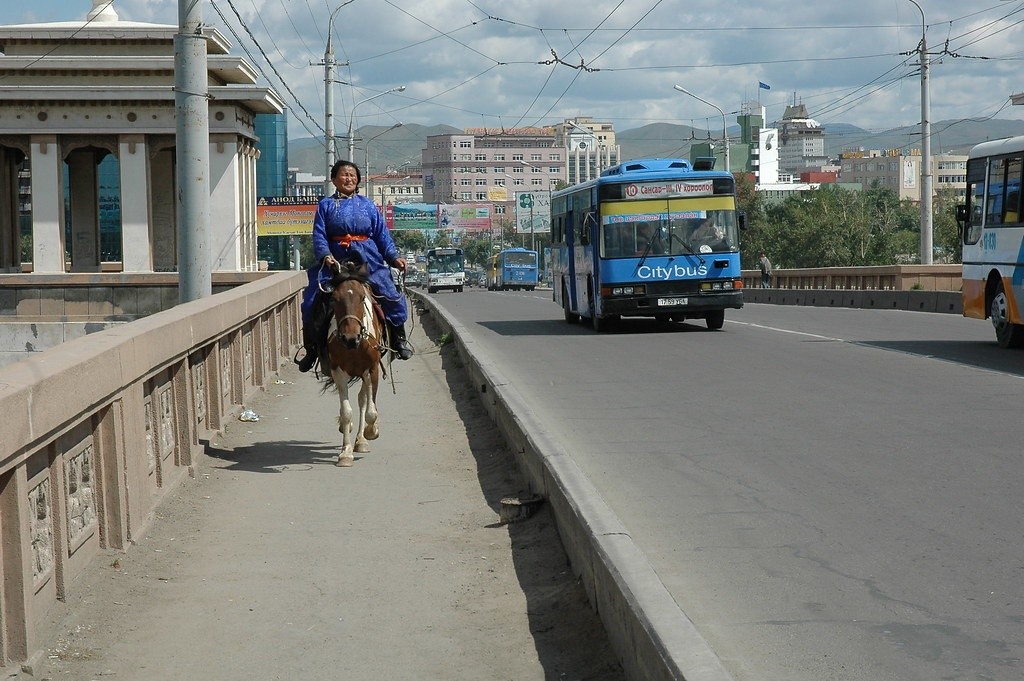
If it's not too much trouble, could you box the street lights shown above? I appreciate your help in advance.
[500,184,524,248]
[472,199,492,257]
[505,173,535,251]
[520,160,551,195]
[482,196,503,252]
[382,176,411,225]
[364,122,403,200]
[674,85,731,240]
[568,121,604,178]
[346,85,406,166]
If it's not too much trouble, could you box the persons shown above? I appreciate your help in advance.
[297,160,413,374]
[637,214,723,254]
[758,251,771,288]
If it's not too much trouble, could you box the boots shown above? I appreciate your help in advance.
[390,322,412,360]
[299,323,319,372]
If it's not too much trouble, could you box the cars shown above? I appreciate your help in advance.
[395,246,428,290]
[464,268,487,288]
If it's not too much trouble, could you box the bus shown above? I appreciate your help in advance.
[550,159,745,335]
[495,248,539,292]
[425,247,467,295]
[951,133,1024,350]
[486,253,500,291]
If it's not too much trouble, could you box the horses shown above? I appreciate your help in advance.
[316,262,390,466]
